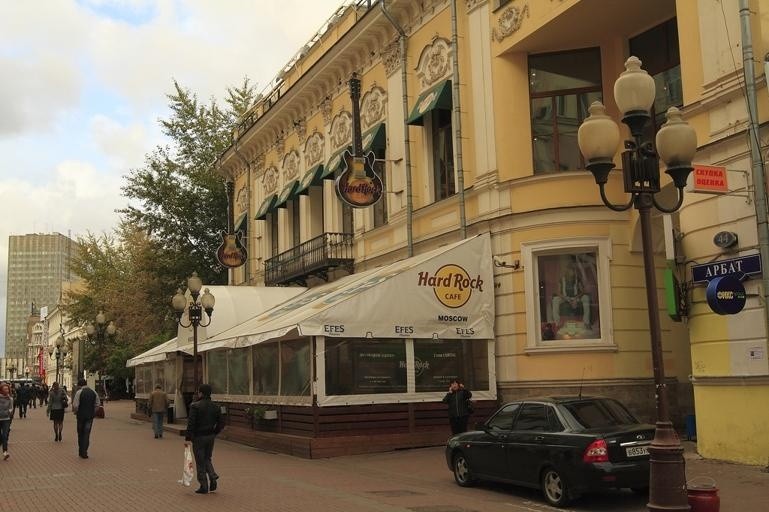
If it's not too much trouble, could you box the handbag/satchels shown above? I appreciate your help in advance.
[467,400,474,414]
[63,399,68,408]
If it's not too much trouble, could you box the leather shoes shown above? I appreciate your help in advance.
[210,480,217,491]
[195,489,208,493]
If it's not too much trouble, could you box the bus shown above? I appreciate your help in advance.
[1,379,34,390]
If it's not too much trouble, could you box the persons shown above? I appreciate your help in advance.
[552,262,592,330]
[443,376,472,435]
[1,380,172,464]
[184,383,227,495]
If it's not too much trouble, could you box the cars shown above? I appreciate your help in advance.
[445,368,658,506]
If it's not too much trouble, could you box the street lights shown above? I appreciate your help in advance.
[579,51,699,511]
[7,363,18,379]
[48,330,71,387]
[86,310,117,408]
[171,271,215,406]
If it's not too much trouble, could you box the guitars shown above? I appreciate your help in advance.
[334,72,383,208]
[215,181,247,267]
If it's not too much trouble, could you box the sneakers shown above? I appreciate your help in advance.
[3,451,9,460]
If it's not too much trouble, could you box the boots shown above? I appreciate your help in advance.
[54,432,58,441]
[59,432,62,441]
